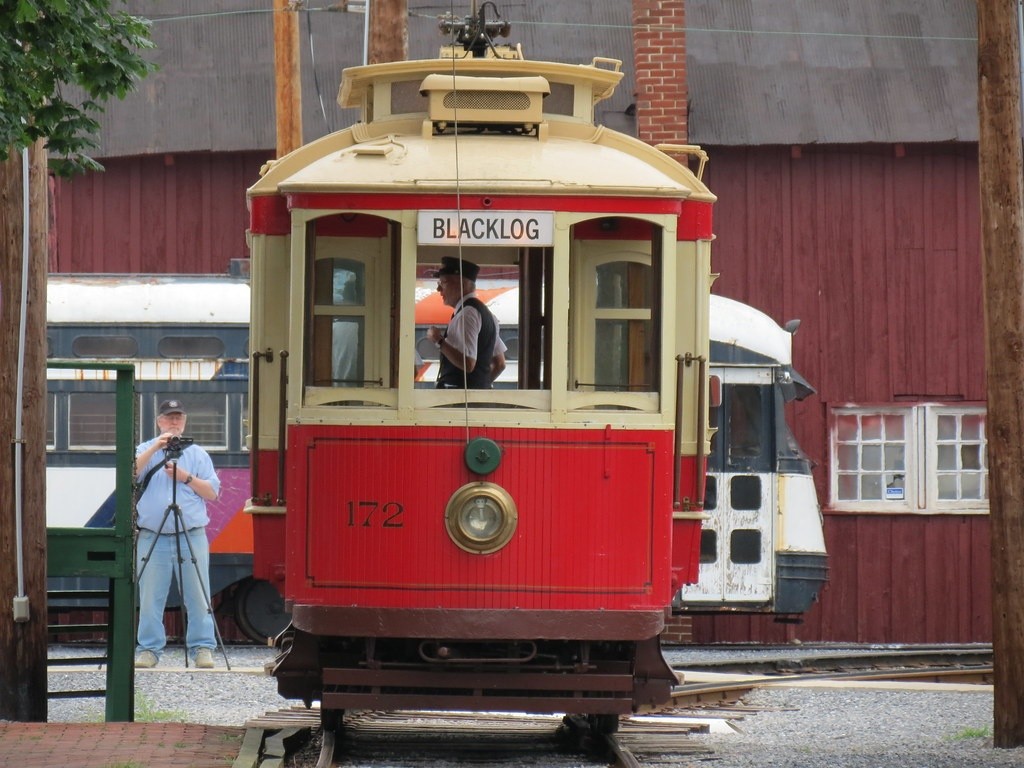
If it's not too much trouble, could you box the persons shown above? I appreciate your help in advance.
[431,256,509,390]
[136,398,222,668]
[332,277,424,388]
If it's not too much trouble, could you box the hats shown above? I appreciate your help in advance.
[432,257,480,282]
[157,400,186,415]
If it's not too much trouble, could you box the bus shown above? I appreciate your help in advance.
[48,255,837,648]
[234,1,726,743]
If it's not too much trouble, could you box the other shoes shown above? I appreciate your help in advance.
[194,648,215,668]
[135,651,159,668]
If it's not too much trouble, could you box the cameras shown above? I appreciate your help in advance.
[167,435,193,448]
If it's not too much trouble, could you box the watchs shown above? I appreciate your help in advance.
[435,338,445,349]
[184,473,193,485]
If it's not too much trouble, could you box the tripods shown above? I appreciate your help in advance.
[98,448,232,672]
